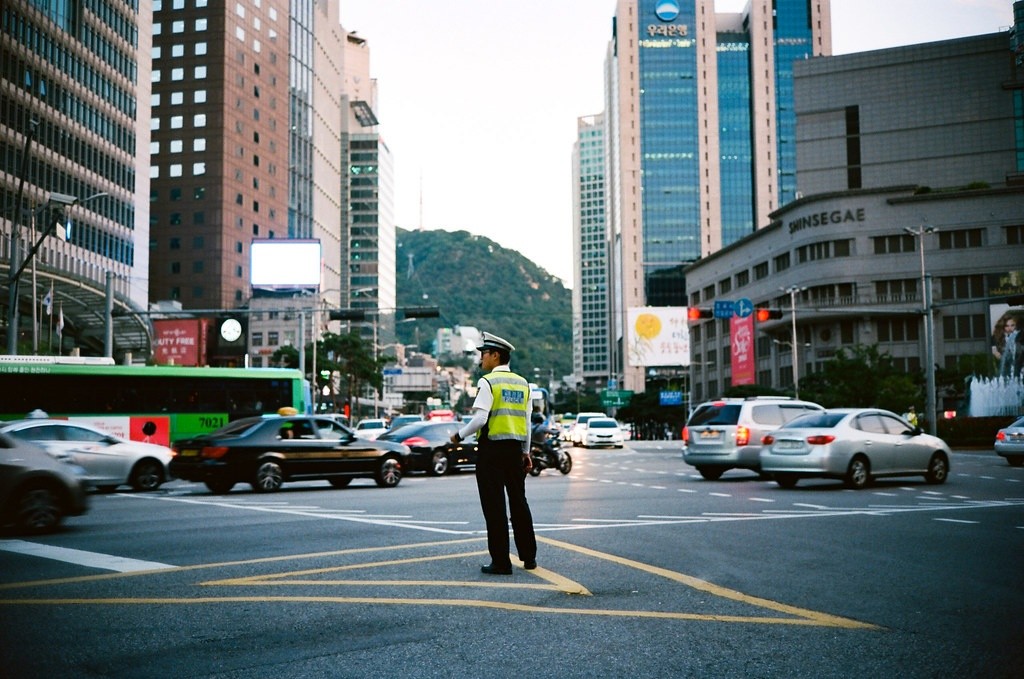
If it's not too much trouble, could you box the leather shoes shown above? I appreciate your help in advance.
[524,559,537,569]
[481,563,512,574]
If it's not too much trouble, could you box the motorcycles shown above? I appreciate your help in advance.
[528,431,572,477]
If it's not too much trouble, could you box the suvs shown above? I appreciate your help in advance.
[680,397,826,479]
[390,414,423,432]
[572,413,607,447]
[354,418,388,440]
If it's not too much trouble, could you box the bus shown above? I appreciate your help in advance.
[530,383,553,433]
[0,354,305,453]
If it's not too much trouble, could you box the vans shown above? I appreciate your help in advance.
[315,414,349,437]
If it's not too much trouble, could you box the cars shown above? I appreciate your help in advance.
[0,434,89,536]
[581,418,624,449]
[169,416,410,495]
[759,407,953,491]
[375,420,479,476]
[994,417,1024,465]
[0,419,176,491]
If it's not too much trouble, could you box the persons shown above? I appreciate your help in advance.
[991,317,1024,377]
[532,412,561,469]
[906,406,917,429]
[450,331,538,575]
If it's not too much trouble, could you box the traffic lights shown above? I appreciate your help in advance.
[688,309,713,319]
[756,310,782,320]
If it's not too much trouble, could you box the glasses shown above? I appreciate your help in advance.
[481,350,490,358]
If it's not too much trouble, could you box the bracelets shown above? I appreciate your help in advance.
[454,433,464,443]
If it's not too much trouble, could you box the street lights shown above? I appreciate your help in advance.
[8,190,108,355]
[779,285,807,399]
[904,223,940,368]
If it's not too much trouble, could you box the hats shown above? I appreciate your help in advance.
[476,332,515,353]
